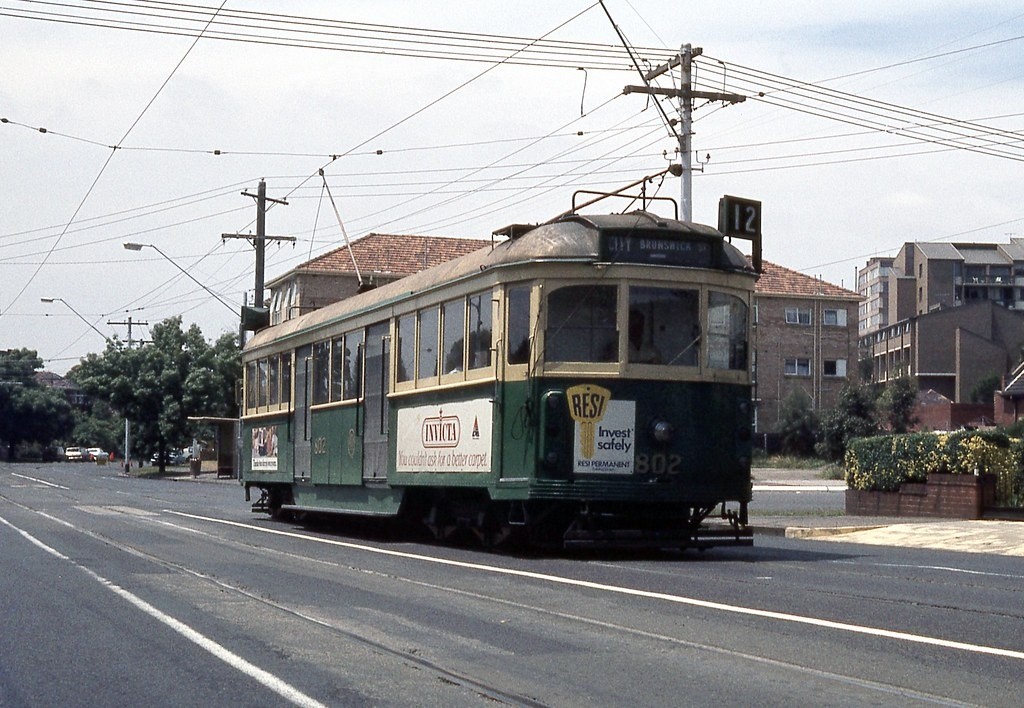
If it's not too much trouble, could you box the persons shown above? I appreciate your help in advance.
[605,309,663,365]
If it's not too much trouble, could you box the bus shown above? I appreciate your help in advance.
[236,166,766,554]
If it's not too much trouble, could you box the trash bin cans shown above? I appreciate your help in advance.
[96,452,108,466]
[190,458,201,475]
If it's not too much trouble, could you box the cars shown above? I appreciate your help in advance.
[46,444,109,464]
[150,445,202,467]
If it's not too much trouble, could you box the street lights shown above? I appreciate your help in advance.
[39,297,132,473]
[122,242,266,336]
